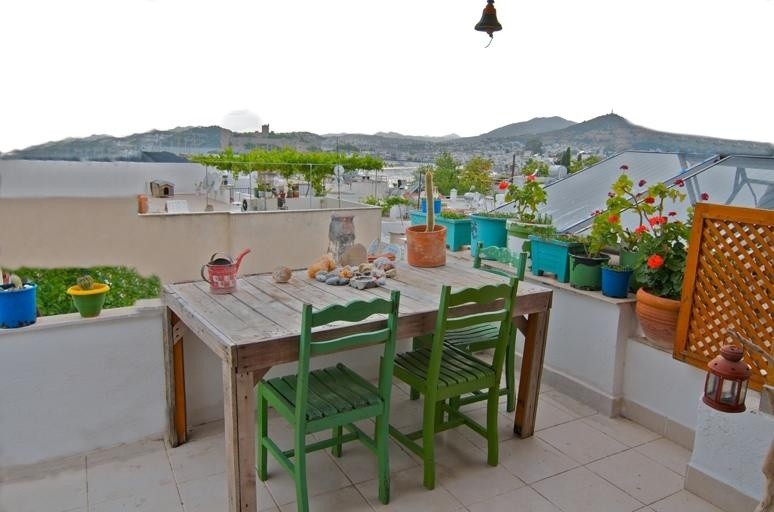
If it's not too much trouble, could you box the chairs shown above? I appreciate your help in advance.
[410,241,528,413]
[380,274,516,490]
[256,287,401,511]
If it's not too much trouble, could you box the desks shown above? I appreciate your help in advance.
[158,257,556,511]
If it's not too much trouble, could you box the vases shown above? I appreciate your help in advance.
[600,265,634,298]
[633,286,680,353]
[409,209,442,229]
[468,209,508,261]
[569,250,610,291]
[505,218,553,266]
[619,241,650,293]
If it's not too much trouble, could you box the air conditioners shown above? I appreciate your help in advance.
[242,197,257,212]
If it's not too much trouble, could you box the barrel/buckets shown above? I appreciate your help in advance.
[0,283,36,329]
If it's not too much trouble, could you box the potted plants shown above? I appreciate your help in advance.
[527,226,584,284]
[66,274,110,318]
[405,171,447,268]
[0,275,37,328]
[435,209,472,252]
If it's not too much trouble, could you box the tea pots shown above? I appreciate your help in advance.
[200,249,251,295]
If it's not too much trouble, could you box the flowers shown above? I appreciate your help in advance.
[588,163,710,297]
[498,174,548,224]
